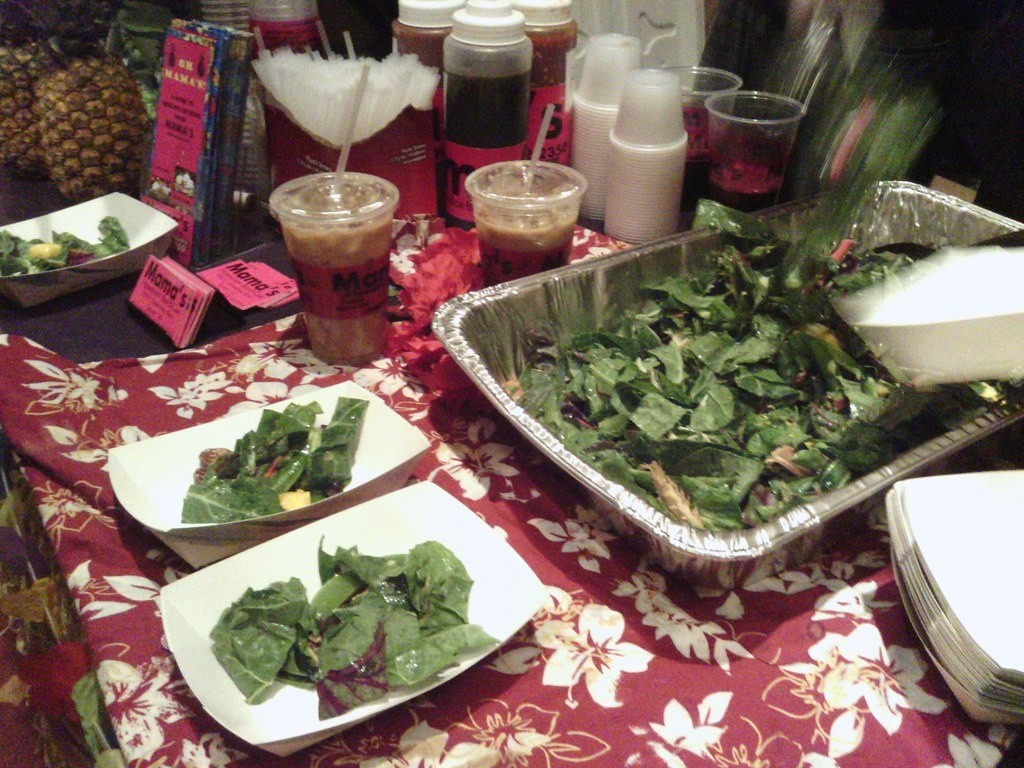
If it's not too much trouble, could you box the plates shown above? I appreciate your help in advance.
[430,180,1024,589]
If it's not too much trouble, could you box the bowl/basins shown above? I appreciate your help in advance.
[159,481,547,758]
[839,245,1024,386]
[0,191,180,308]
[108,379,431,570]
[885,471,1024,725]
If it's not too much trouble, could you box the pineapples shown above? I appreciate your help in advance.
[0,0,147,205]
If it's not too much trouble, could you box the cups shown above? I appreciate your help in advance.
[705,90,808,213]
[666,65,744,213]
[464,161,588,288]
[268,172,394,366]
[250,0,322,56]
[569,33,688,244]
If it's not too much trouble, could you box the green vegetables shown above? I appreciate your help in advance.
[182,396,371,519]
[0,216,128,277]
[208,529,498,719]
[526,197,1003,519]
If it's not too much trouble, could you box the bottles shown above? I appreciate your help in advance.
[513,0,579,163]
[440,0,533,233]
[391,0,466,217]
